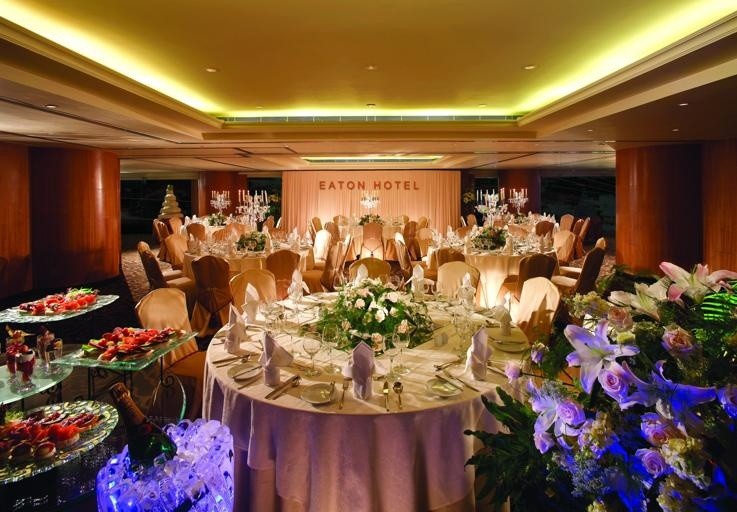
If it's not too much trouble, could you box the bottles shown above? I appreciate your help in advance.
[108,382,218,512]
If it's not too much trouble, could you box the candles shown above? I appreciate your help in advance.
[493,189,494,195]
[228,191,229,198]
[212,191,213,199]
[247,191,249,202]
[265,190,266,199]
[510,189,511,198]
[243,190,245,206]
[214,191,216,198]
[223,191,224,195]
[238,190,241,205]
[477,190,478,202]
[503,188,504,199]
[225,191,226,198]
[486,189,488,194]
[526,189,527,198]
[255,191,257,201]
[261,190,263,201]
[501,189,502,200]
[266,194,267,203]
[480,190,482,201]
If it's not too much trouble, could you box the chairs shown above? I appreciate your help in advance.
[301,245,337,293]
[212,229,233,242]
[456,228,472,239]
[574,217,590,258]
[556,234,575,266]
[424,248,466,281]
[493,220,505,228]
[313,230,332,262]
[551,247,605,297]
[535,221,554,244]
[190,255,233,336]
[337,241,342,285]
[395,215,409,226]
[560,214,574,231]
[515,277,559,329]
[436,261,480,300]
[266,249,301,300]
[308,223,316,243]
[573,218,584,235]
[153,218,172,262]
[381,226,401,260]
[135,288,207,426]
[186,223,206,243]
[334,215,348,225]
[397,215,409,224]
[467,215,477,226]
[558,237,607,279]
[349,257,392,281]
[315,234,352,285]
[507,224,529,241]
[135,240,193,287]
[361,222,383,261]
[228,269,278,314]
[415,216,431,256]
[404,221,422,262]
[169,217,183,233]
[501,253,556,302]
[324,222,341,246]
[460,216,466,228]
[165,234,188,270]
[312,217,322,232]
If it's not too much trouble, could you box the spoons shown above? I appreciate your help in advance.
[392,382,405,411]
[271,378,299,399]
[224,357,250,369]
[429,361,455,369]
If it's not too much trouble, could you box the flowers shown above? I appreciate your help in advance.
[302,277,434,356]
[464,262,737,512]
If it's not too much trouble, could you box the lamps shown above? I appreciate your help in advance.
[475,195,509,221]
[210,194,231,215]
[508,192,529,214]
[236,196,271,232]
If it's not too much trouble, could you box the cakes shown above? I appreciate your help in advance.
[0,423,81,463]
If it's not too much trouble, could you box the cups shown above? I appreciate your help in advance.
[45,338,63,376]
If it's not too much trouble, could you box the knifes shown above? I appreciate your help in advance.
[459,378,484,393]
[383,380,390,412]
[210,349,256,365]
[263,371,300,399]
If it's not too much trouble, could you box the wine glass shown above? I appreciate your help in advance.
[243,240,252,256]
[5,343,24,385]
[288,285,301,313]
[15,349,37,391]
[207,236,216,256]
[36,333,53,369]
[417,279,429,296]
[303,331,322,376]
[323,325,338,369]
[455,313,469,353]
[333,276,346,296]
[283,314,299,354]
[431,282,443,303]
[390,276,400,291]
[378,274,387,287]
[270,300,283,334]
[260,301,275,332]
[383,337,398,376]
[392,327,411,370]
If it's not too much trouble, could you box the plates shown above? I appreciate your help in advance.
[494,334,530,353]
[425,376,465,397]
[299,384,336,402]
[275,295,318,310]
[229,362,260,379]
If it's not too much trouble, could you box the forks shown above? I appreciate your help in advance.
[337,379,352,410]
[434,370,460,392]
[487,335,525,346]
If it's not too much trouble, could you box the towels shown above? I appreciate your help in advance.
[306,231,312,247]
[547,229,552,246]
[287,268,310,298]
[460,273,475,303]
[541,211,546,221]
[504,235,514,253]
[225,303,246,352]
[461,234,471,253]
[471,222,478,236]
[197,237,203,253]
[445,224,454,240]
[499,311,513,336]
[290,226,300,252]
[538,234,545,252]
[258,333,293,385]
[351,341,375,399]
[183,215,188,224]
[466,327,493,380]
[192,215,198,224]
[187,233,193,252]
[265,234,274,252]
[240,282,263,322]
[405,263,434,297]
[546,215,551,222]
[355,264,368,284]
[227,237,238,256]
[551,215,555,224]
